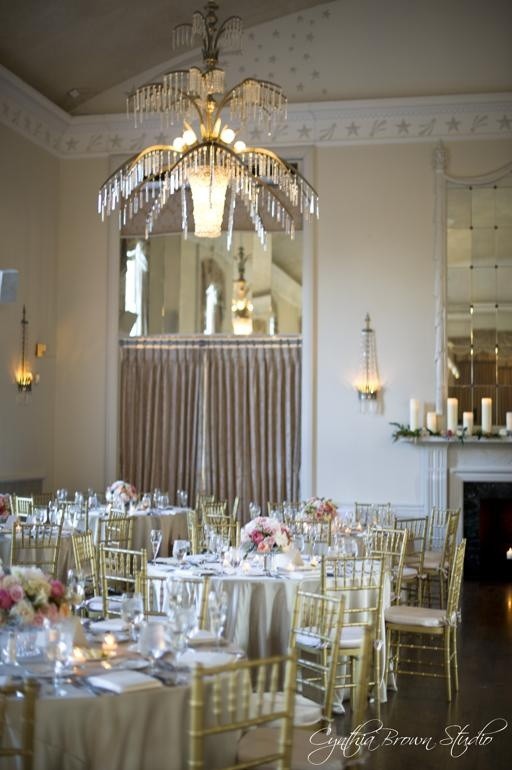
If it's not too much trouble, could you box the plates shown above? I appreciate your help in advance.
[90,620,131,633]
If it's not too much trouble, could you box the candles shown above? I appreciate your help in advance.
[407,397,511,436]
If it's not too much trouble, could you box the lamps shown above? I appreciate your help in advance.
[352,314,384,403]
[96,0,321,251]
[231,246,254,336]
[12,304,34,392]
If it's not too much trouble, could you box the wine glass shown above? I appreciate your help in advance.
[44,621,76,693]
[150,525,356,577]
[120,590,228,675]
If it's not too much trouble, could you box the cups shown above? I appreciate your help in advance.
[55,487,188,514]
[30,506,47,526]
[248,501,296,525]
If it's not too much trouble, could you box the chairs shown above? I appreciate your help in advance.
[0,491,467,770]
